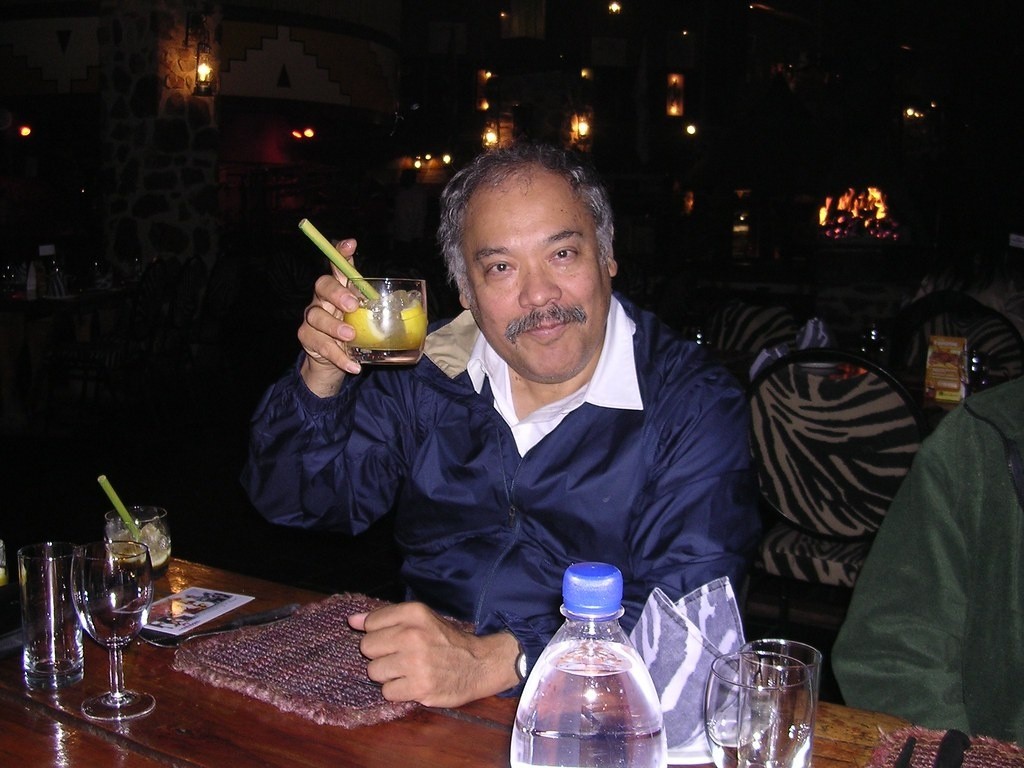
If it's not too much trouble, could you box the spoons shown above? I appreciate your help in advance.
[137,601,303,649]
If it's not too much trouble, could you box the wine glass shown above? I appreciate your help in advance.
[70,539,154,722]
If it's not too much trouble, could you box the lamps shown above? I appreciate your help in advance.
[183,1,214,100]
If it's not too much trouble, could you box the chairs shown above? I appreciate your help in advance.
[711,294,1023,641]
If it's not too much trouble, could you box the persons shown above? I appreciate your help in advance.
[240,139,762,709]
[391,169,426,258]
[831,258,1024,741]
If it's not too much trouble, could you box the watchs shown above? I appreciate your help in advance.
[501,628,527,680]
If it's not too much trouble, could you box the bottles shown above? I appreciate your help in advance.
[510,562,669,768]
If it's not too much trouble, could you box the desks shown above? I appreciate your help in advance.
[0,552,1024,768]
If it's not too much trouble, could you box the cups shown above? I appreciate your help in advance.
[342,277,428,365]
[736,637,822,768]
[0,539,9,588]
[17,540,87,693]
[859,332,891,378]
[704,651,812,768]
[957,349,991,398]
[103,504,172,580]
